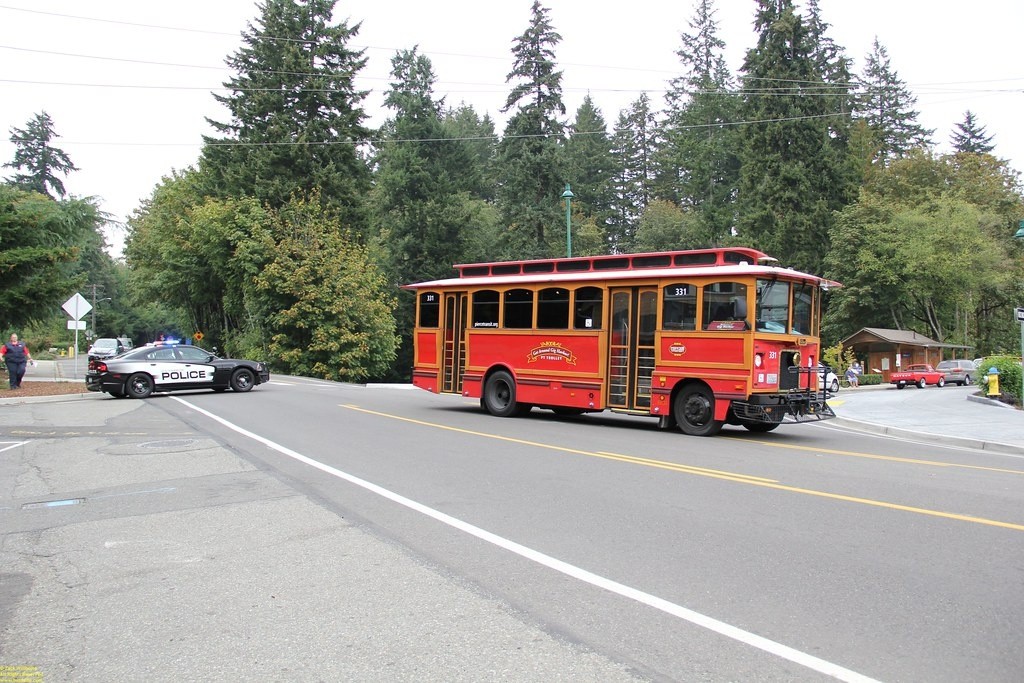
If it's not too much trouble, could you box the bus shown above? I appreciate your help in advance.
[397,248,840,440]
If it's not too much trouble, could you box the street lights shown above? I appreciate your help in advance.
[561,183,575,258]
[91,284,111,337]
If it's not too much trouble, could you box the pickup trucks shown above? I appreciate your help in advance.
[890,363,947,388]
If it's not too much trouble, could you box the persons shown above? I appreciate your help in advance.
[0,333,34,390]
[845,367,859,388]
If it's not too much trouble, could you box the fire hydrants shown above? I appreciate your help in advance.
[985,369,1003,396]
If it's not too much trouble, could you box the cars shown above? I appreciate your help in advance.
[817,360,841,394]
[937,358,979,385]
[88,338,133,361]
[86,343,269,398]
